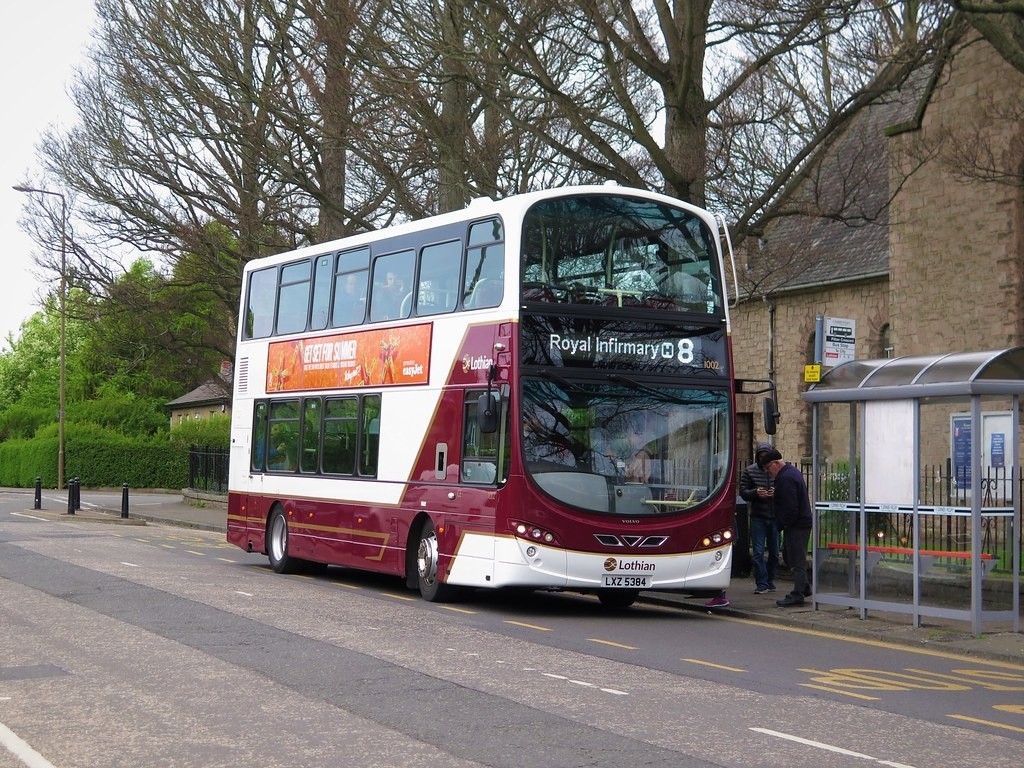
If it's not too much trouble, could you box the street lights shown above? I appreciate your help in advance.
[14,184,66,490]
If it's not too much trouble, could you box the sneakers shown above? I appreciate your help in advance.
[766,581,776,592]
[801,583,812,597]
[776,592,804,607]
[753,585,769,594]
[704,597,729,606]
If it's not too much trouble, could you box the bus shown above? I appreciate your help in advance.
[227,180,781,606]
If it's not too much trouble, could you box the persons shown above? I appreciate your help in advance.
[760,450,813,607]
[333,269,409,327]
[257,424,288,469]
[738,441,779,594]
[606,423,656,500]
[703,590,729,608]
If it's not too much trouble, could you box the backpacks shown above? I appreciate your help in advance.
[635,449,661,484]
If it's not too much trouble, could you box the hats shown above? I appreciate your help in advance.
[757,448,770,453]
[757,449,782,468]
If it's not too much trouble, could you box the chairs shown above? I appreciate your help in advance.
[523,287,678,311]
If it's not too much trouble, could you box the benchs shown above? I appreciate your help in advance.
[817,544,1002,575]
[360,278,503,318]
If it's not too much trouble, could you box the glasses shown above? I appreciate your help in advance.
[763,462,773,472]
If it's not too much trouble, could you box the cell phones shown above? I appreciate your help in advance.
[768,490,773,493]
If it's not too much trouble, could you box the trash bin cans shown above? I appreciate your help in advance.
[731,495,751,579]
